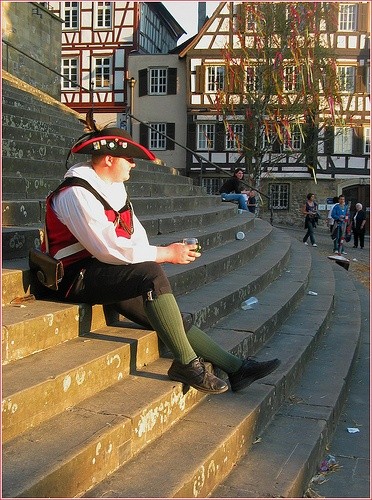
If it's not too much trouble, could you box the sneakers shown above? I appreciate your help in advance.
[332,250,339,254]
[341,252,348,255]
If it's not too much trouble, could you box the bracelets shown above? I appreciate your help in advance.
[362,223,364,226]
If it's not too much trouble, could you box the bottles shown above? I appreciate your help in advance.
[341,210,345,221]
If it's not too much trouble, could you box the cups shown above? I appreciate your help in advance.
[312,292,317,295]
[238,209,242,214]
[236,231,245,240]
[182,238,199,253]
[240,296,258,311]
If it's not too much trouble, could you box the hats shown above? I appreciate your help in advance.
[71,127,157,161]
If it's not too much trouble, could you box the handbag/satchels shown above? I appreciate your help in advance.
[29,248,62,291]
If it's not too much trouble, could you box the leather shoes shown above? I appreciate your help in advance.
[228,357,281,392]
[167,356,229,393]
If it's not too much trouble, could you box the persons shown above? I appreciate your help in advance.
[348,202,367,249]
[239,186,257,214]
[301,192,323,247]
[327,194,351,255]
[40,127,282,395]
[218,167,250,211]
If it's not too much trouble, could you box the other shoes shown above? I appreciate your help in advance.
[304,242,308,245]
[360,246,363,249]
[313,244,317,247]
[352,246,358,248]
